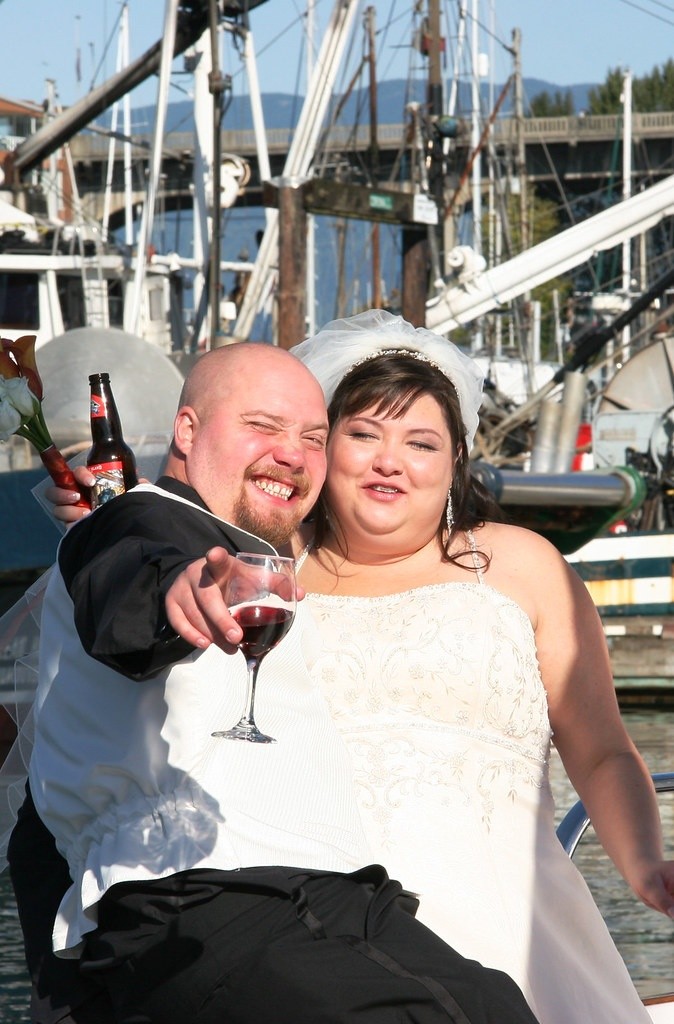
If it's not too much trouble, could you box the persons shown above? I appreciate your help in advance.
[7,341,541,1024]
[44,309,674,1024]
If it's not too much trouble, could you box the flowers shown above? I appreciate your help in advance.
[0,335,87,511]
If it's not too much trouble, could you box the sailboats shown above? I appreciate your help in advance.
[3,1,669,631]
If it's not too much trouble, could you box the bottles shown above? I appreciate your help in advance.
[81,373,139,514]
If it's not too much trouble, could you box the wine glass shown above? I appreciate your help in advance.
[208,552,298,747]
